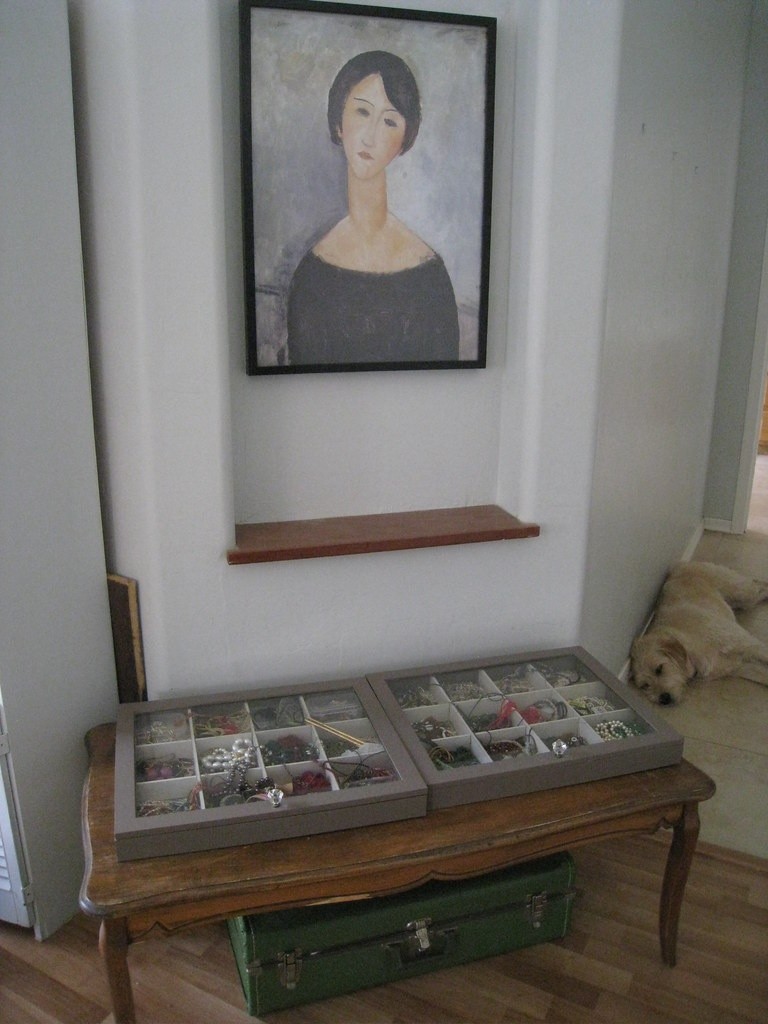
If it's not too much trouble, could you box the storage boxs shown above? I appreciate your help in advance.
[363,647,686,810]
[114,678,431,861]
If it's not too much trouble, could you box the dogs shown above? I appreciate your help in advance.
[630,561,768,707]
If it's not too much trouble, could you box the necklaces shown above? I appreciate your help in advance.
[132,688,395,820]
[395,654,650,773]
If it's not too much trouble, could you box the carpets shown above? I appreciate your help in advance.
[626,530,768,871]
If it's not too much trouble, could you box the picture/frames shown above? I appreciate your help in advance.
[238,0,498,375]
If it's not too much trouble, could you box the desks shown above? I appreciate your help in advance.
[78,723,715,1024]
